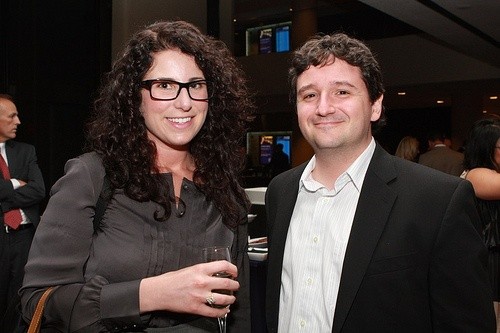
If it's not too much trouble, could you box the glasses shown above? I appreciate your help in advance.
[140,79,215,101]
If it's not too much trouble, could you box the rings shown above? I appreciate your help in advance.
[206,292,213,304]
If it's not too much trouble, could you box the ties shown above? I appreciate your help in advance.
[0,154,22,230]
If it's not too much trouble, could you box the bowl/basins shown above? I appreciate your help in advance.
[247,247,268,261]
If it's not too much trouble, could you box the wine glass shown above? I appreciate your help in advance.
[200,245,233,333]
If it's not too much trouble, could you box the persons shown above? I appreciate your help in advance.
[395,112,500,321]
[18,20,252,333]
[0,93,47,333]
[265,143,292,186]
[264,34,496,333]
